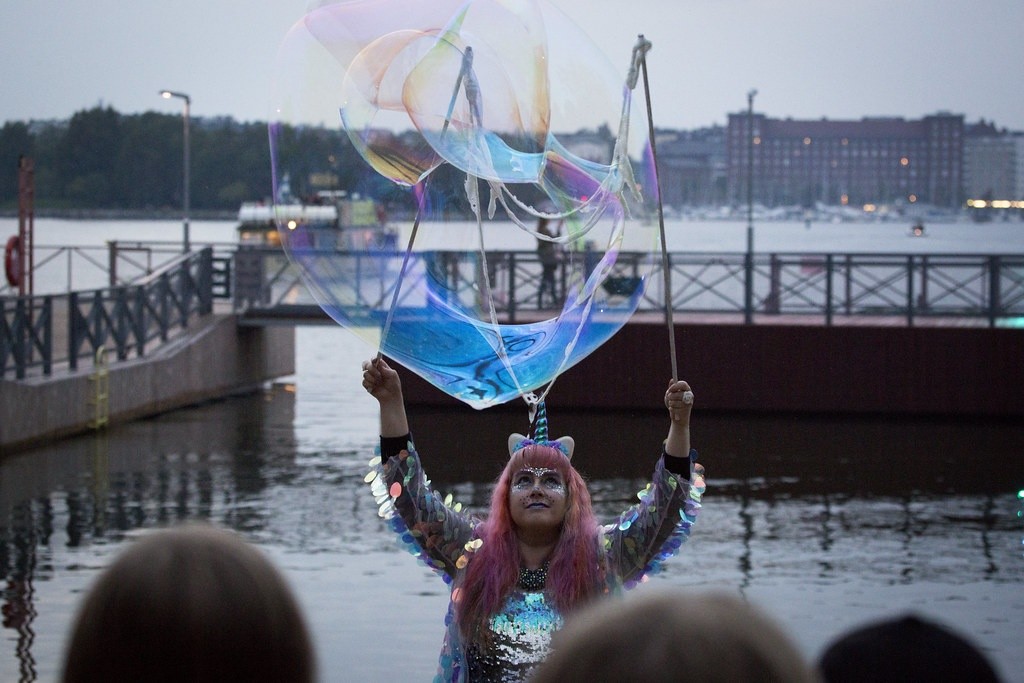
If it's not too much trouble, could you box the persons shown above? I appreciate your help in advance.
[537,217,564,311]
[361,357,703,683]
[531,586,805,683]
[61,523,315,683]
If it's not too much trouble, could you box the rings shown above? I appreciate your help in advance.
[683,391,693,404]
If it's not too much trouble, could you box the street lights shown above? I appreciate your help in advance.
[160,89,190,254]
